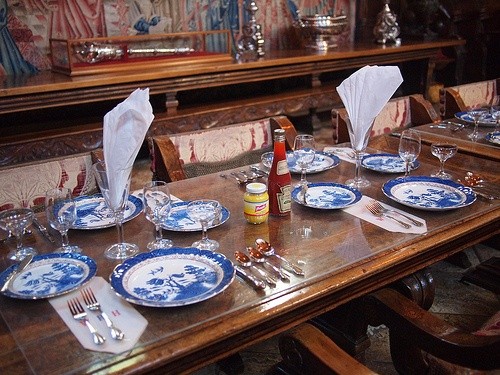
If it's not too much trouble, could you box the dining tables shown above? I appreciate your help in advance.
[0,106,500,375]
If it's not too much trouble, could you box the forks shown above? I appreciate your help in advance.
[66,287,124,344]
[365,199,424,229]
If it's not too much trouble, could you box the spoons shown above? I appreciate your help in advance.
[465,171,500,198]
[232,238,305,285]
[222,169,263,184]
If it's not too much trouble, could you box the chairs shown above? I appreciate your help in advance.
[439,78,500,118]
[330,94,440,144]
[254,285,500,375]
[0,151,105,214]
[152,115,306,183]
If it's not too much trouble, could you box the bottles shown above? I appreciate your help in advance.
[243,183,270,224]
[268,128,291,217]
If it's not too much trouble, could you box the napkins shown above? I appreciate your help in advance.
[335,66,404,150]
[101,88,153,206]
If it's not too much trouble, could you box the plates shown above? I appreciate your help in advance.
[160,201,230,230]
[285,150,338,173]
[381,177,477,211]
[454,108,500,127]
[0,253,96,300]
[109,245,235,308]
[53,193,145,231]
[291,182,362,210]
[360,153,420,172]
[485,133,500,145]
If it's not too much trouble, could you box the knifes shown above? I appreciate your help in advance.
[32,218,55,244]
[1,254,33,293]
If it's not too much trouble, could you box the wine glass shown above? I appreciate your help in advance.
[466,108,488,138]
[0,159,222,252]
[261,118,457,186]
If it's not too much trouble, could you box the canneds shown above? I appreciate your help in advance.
[243,183,269,224]
